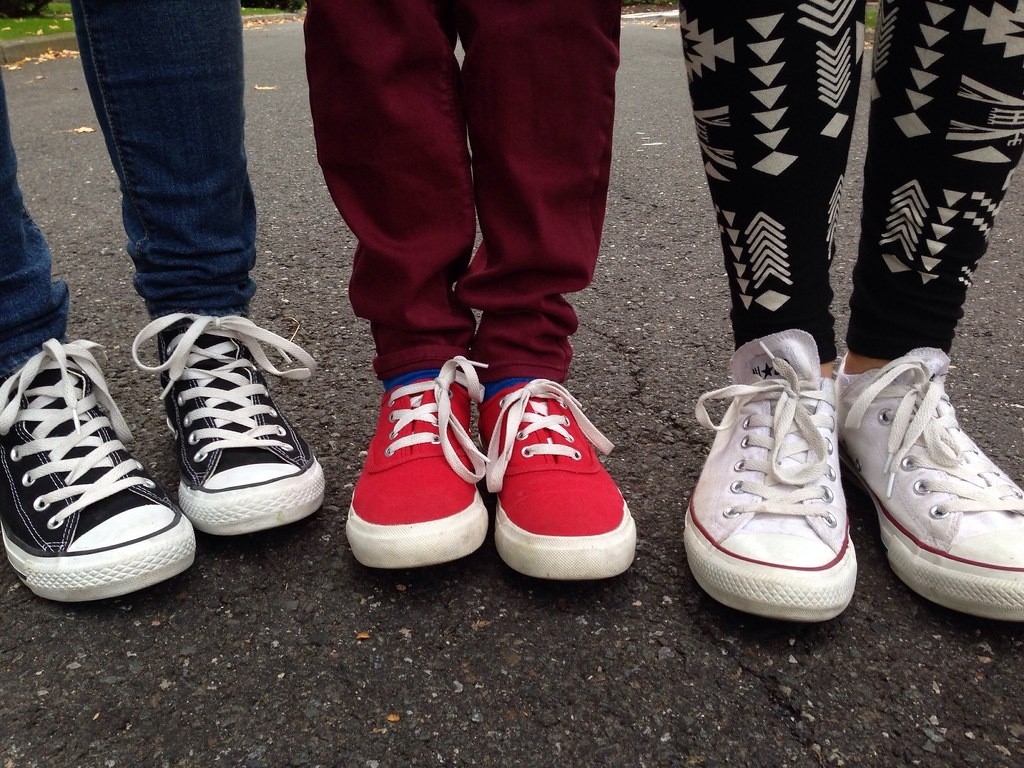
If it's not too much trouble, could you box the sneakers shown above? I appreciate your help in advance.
[834,352,1024,622]
[346,355,492,569]
[477,378,637,581]
[131,312,325,536]
[0,337,196,603]
[684,328,858,622]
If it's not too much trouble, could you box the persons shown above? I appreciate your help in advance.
[303,0,637,582]
[0,0,324,603]
[677,0,1024,623]
